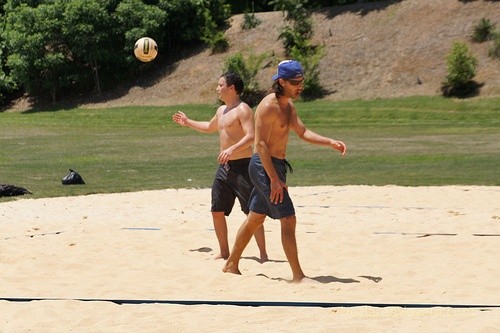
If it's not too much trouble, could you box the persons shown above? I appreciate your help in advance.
[221,59,347,286]
[172,71,270,265]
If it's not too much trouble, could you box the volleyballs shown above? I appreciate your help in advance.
[134,37,158,62]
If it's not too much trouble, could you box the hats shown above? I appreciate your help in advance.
[272,60,304,81]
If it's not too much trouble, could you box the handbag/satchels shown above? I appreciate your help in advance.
[62,169,85,185]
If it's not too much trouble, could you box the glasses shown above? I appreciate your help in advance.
[283,78,305,85]
